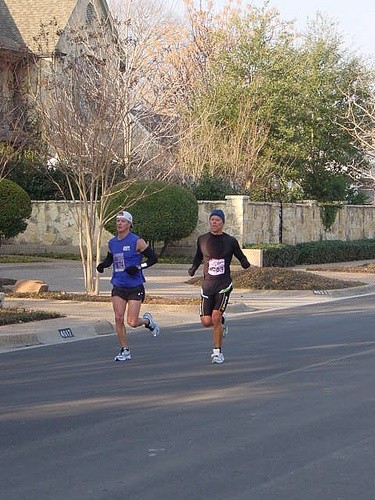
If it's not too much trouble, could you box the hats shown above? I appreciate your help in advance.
[209,210,225,224]
[116,211,133,228]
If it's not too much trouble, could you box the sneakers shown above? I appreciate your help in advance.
[211,349,224,364]
[222,312,228,337]
[143,312,160,337]
[113,347,131,361]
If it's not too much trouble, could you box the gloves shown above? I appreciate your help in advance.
[97,264,103,273]
[124,265,139,276]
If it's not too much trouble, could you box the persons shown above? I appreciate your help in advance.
[188,209,250,364]
[97,211,160,362]
